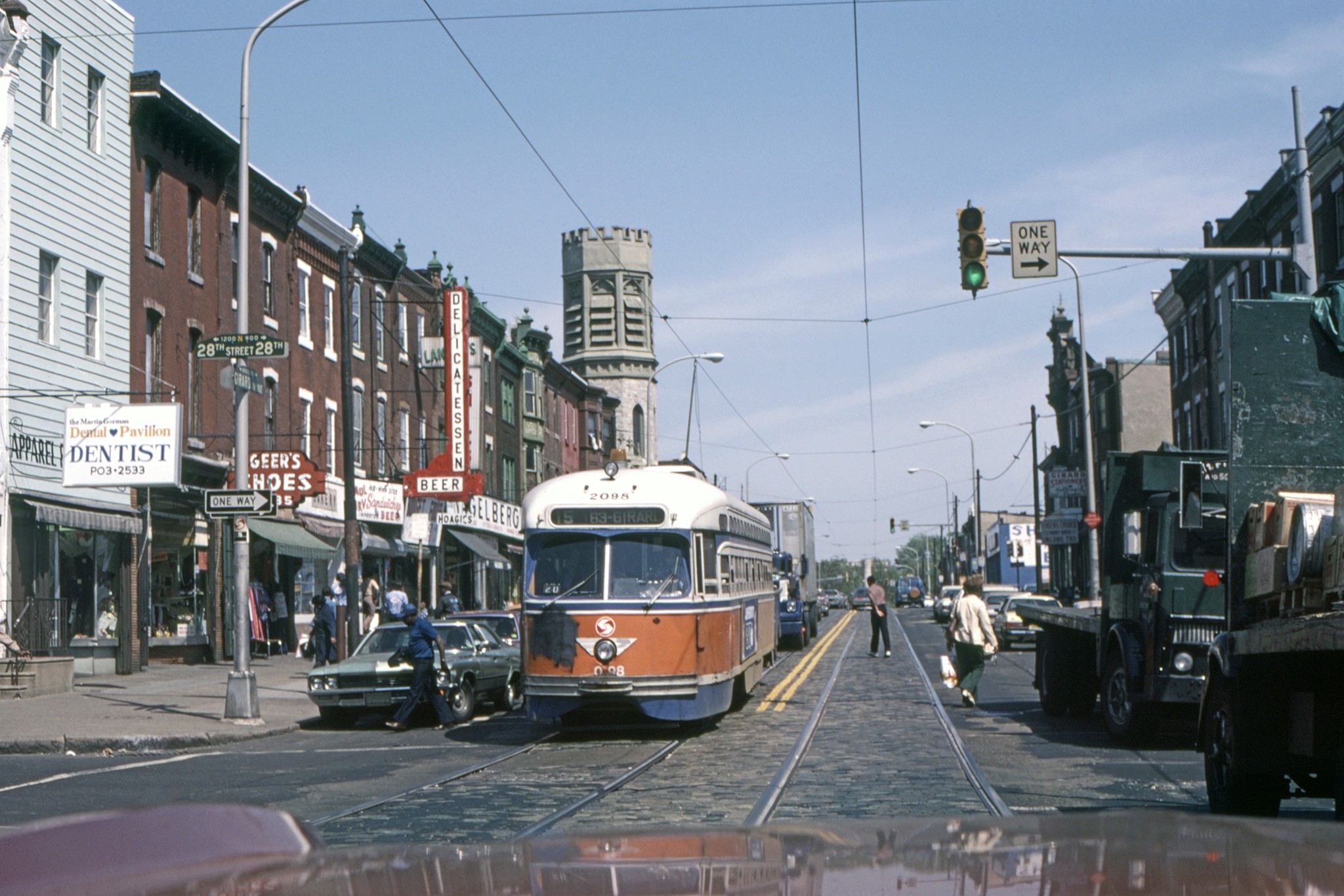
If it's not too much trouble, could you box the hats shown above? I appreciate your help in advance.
[962,574,983,589]
[396,603,417,618]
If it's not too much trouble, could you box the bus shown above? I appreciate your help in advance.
[517,359,782,727]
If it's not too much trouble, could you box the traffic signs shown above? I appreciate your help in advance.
[1009,220,1058,279]
[204,489,272,514]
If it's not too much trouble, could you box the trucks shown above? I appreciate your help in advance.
[1195,296,1343,820]
[1014,439,1229,748]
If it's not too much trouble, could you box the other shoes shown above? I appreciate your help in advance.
[433,721,454,731]
[962,690,976,707]
[386,720,406,732]
[885,651,891,657]
[866,650,878,657]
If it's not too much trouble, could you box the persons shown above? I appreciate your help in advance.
[950,575,998,707]
[865,576,891,657]
[420,602,428,619]
[267,578,288,654]
[362,570,379,634]
[311,587,337,668]
[385,604,455,730]
[331,573,347,606]
[386,579,409,622]
[435,581,464,619]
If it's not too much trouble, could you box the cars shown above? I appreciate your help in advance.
[983,590,1038,627]
[933,585,962,624]
[1023,582,1037,594]
[994,592,1063,652]
[444,609,521,649]
[815,588,849,621]
[307,618,521,728]
[982,584,1019,598]
[850,586,872,609]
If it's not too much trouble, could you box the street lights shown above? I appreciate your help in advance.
[907,468,955,586]
[918,420,979,573]
[982,238,1100,601]
[646,352,725,466]
[894,546,920,578]
[746,453,790,504]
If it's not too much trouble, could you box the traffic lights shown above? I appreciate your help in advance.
[956,206,989,289]
[890,518,895,533]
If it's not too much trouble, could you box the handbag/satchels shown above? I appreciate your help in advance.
[940,654,958,689]
[945,628,955,644]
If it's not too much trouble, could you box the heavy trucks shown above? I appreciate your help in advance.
[747,501,818,652]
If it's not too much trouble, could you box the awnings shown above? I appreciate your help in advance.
[23,498,143,535]
[247,518,339,561]
[445,528,512,571]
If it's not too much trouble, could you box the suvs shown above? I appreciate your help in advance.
[895,575,926,607]
